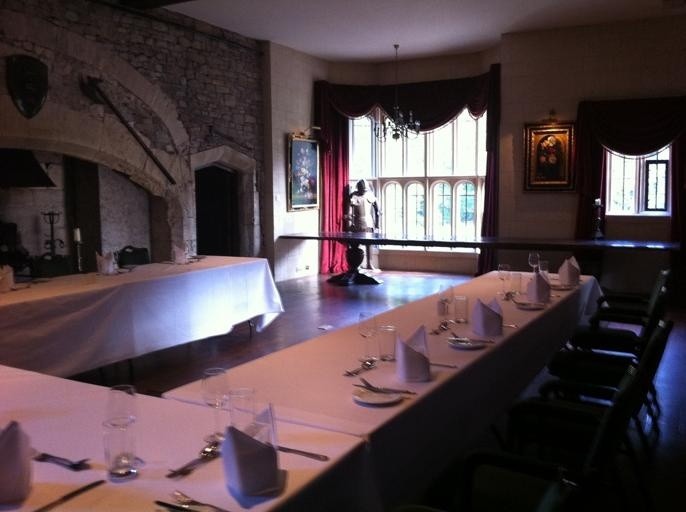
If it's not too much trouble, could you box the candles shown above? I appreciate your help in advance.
[73,228,81,242]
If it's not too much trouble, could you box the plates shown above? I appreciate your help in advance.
[350,387,404,405]
[516,301,545,310]
[547,278,572,290]
[447,337,488,349]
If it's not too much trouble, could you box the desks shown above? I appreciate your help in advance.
[279,231,680,287]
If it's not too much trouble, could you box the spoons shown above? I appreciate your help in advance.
[168,443,219,475]
[342,360,377,379]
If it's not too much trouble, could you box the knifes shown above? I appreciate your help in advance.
[33,479,106,512]
[152,500,200,511]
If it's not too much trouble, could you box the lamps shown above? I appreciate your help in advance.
[369,45,421,141]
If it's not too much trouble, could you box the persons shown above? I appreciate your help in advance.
[343,178,381,272]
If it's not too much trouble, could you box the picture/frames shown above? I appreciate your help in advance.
[286,132,321,211]
[521,119,577,193]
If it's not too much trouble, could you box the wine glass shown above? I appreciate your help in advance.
[200,367,232,444]
[107,384,146,469]
[528,252,539,276]
[437,283,457,328]
[496,264,512,294]
[357,311,378,363]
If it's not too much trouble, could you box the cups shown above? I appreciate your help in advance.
[377,325,398,362]
[538,261,549,277]
[511,273,522,294]
[101,417,137,483]
[454,295,468,325]
[228,385,258,436]
